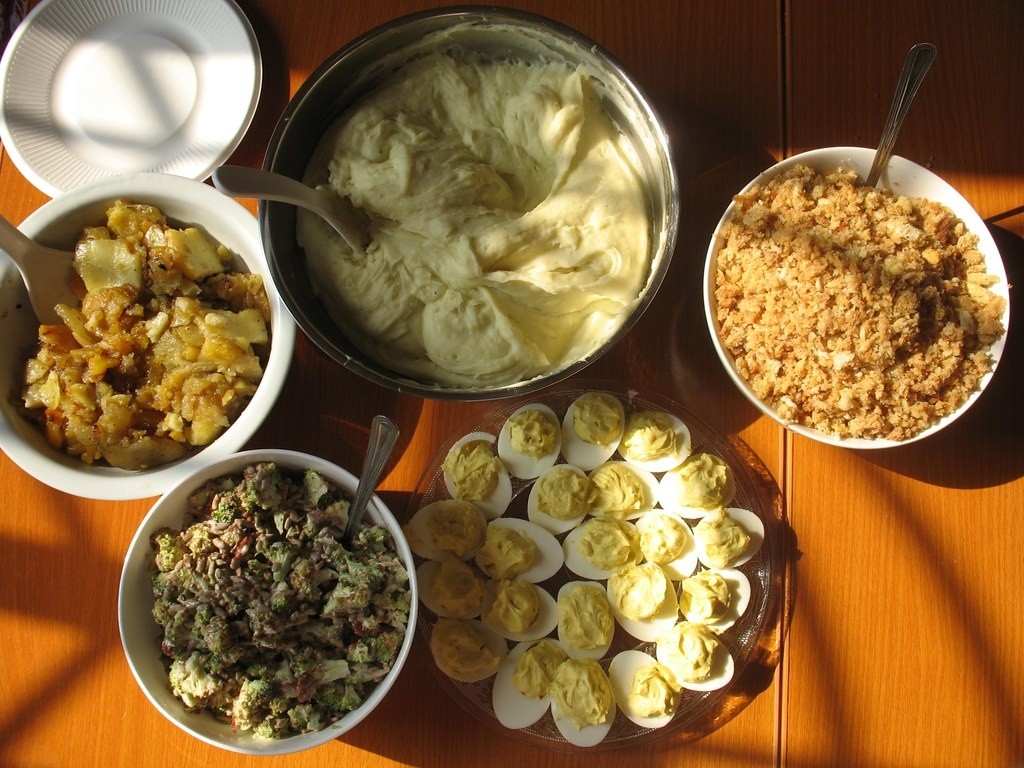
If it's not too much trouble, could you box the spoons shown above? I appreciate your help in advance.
[211,166,372,252]
[0,214,82,326]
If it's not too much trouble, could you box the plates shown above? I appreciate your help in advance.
[408,383,775,740]
[0,0,263,199]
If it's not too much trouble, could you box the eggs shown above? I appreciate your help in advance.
[404,393,766,747]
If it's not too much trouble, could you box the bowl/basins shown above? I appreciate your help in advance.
[0,173,294,500]
[704,147,1010,450]
[258,5,681,403]
[118,448,418,754]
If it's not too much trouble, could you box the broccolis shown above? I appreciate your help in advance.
[147,462,413,739]
